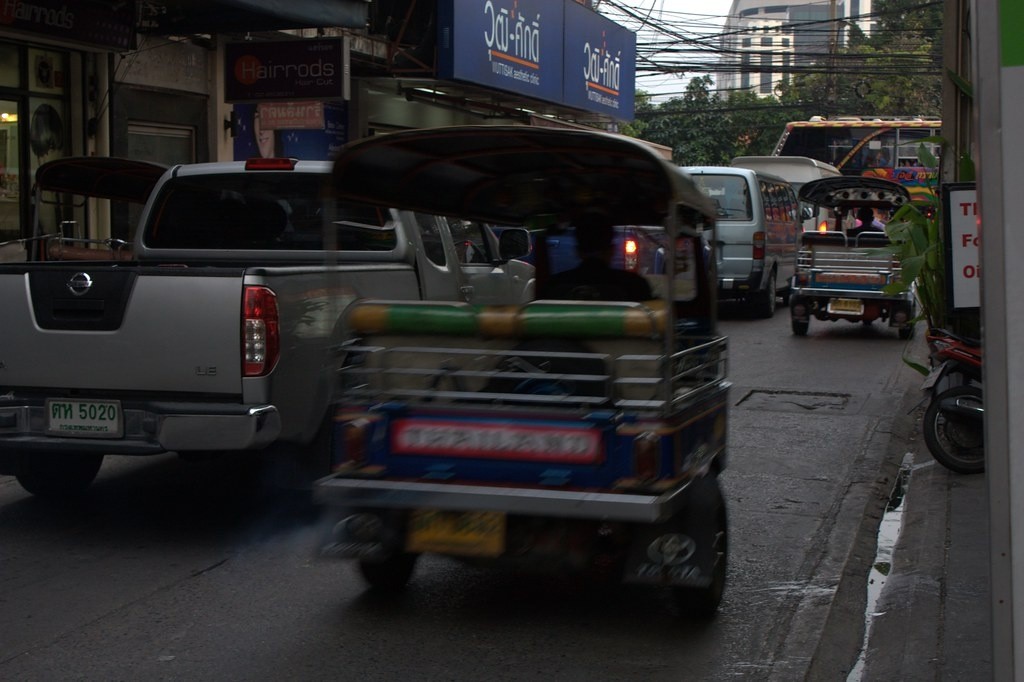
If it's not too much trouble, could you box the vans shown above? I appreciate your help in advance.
[676,165,813,320]
[729,154,845,231]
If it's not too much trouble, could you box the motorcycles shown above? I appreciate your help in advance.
[334,123,733,637]
[920,324,984,474]
[790,176,919,343]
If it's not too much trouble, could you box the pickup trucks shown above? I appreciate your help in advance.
[2,156,533,532]
[488,215,724,341]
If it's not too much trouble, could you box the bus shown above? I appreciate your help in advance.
[770,115,943,223]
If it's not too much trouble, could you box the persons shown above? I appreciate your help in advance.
[852,208,884,234]
[238,107,284,159]
[541,222,652,303]
